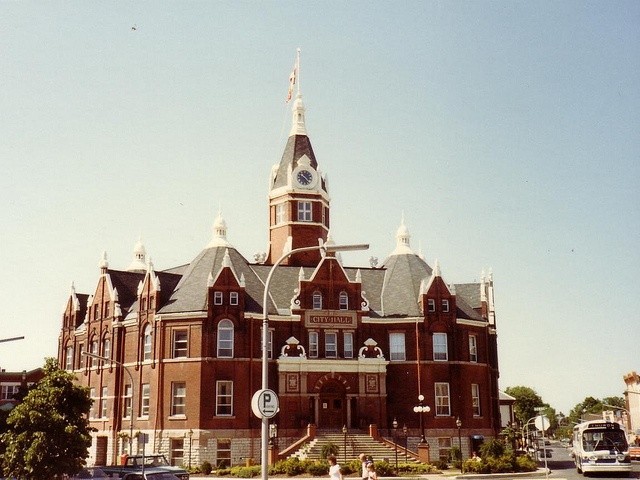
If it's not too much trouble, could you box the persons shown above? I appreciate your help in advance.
[328,456,343,480]
[367,462,377,480]
[360,453,373,480]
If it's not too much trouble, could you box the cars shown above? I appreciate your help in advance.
[70,467,113,480]
[121,469,182,480]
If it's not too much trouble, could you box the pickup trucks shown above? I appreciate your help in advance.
[96,454,190,480]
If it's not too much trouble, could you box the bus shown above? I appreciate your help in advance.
[571,419,633,477]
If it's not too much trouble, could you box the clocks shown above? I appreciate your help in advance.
[291,164,317,189]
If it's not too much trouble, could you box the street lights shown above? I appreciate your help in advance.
[601,403,631,440]
[412,394,431,444]
[0,336,24,343]
[526,414,548,460]
[342,424,348,465]
[522,423,535,452]
[187,427,195,470]
[257,242,370,480]
[402,424,408,464]
[455,416,463,475]
[392,416,398,477]
[81,350,134,457]
